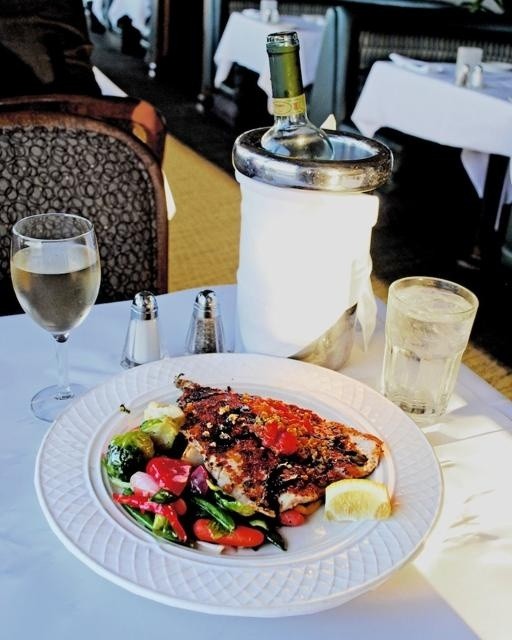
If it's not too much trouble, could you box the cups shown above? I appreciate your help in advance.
[379,276,480,426]
[455,46,484,75]
[232,126,394,373]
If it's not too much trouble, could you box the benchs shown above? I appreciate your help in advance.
[204,0,511,239]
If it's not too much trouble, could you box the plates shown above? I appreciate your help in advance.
[35,352,445,620]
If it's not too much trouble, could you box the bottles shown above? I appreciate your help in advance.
[119,289,169,371]
[455,63,486,91]
[257,32,335,164]
[178,290,226,359]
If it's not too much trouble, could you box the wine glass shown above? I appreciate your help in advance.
[10,212,102,424]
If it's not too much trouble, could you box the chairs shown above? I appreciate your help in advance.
[0,1,168,314]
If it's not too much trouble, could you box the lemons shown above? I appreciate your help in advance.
[324,478,392,524]
